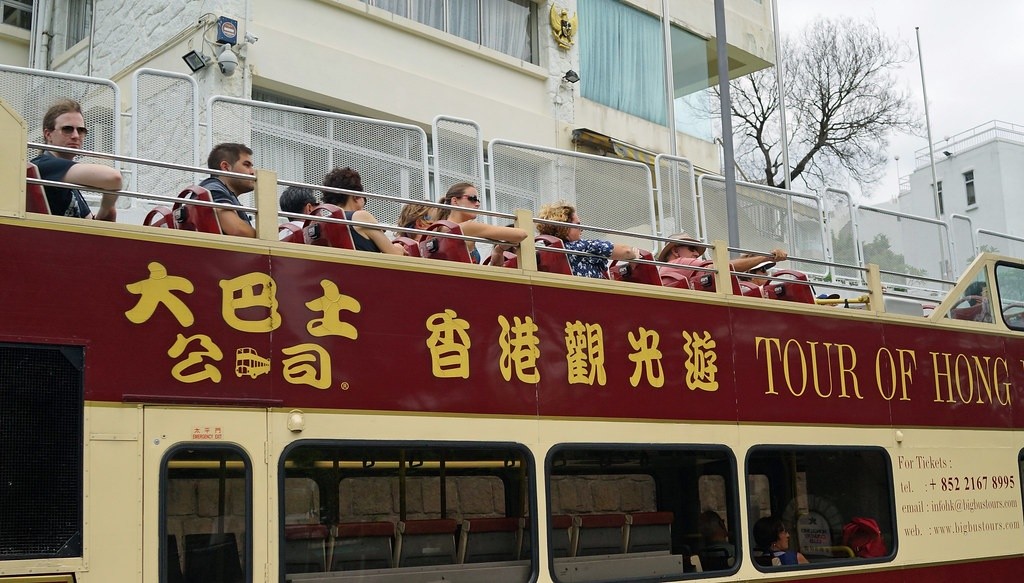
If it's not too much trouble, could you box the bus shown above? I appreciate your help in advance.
[2,98,1023,583]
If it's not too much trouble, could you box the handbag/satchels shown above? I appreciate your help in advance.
[840,516,888,557]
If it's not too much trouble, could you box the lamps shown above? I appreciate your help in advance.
[563,70,581,83]
[943,151,953,156]
[181,51,206,74]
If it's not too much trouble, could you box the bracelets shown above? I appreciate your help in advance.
[498,241,507,253]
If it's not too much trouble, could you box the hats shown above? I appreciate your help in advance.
[659,234,706,263]
[745,260,776,272]
[817,293,840,299]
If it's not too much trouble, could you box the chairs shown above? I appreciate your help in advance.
[740,281,762,298]
[922,305,949,318]
[624,511,783,573]
[382,237,421,257]
[660,272,691,290]
[571,512,629,558]
[950,296,991,322]
[688,260,743,296]
[165,515,571,583]
[142,206,175,229]
[25,162,52,216]
[278,223,305,245]
[418,220,473,264]
[1002,303,1024,328]
[608,249,662,286]
[171,185,223,235]
[763,270,815,304]
[482,250,518,269]
[535,235,573,276]
[301,204,356,250]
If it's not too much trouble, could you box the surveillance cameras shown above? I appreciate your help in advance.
[218,50,239,77]
[244,32,259,44]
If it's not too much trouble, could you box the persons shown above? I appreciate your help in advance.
[748,516,809,568]
[816,293,840,308]
[391,183,528,264]
[187,143,260,239]
[279,166,405,257]
[964,282,997,321]
[30,102,123,223]
[696,509,736,567]
[656,233,787,295]
[736,253,776,300]
[535,205,639,281]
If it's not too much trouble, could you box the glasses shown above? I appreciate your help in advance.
[456,194,481,206]
[676,244,699,253]
[51,126,89,137]
[755,266,767,273]
